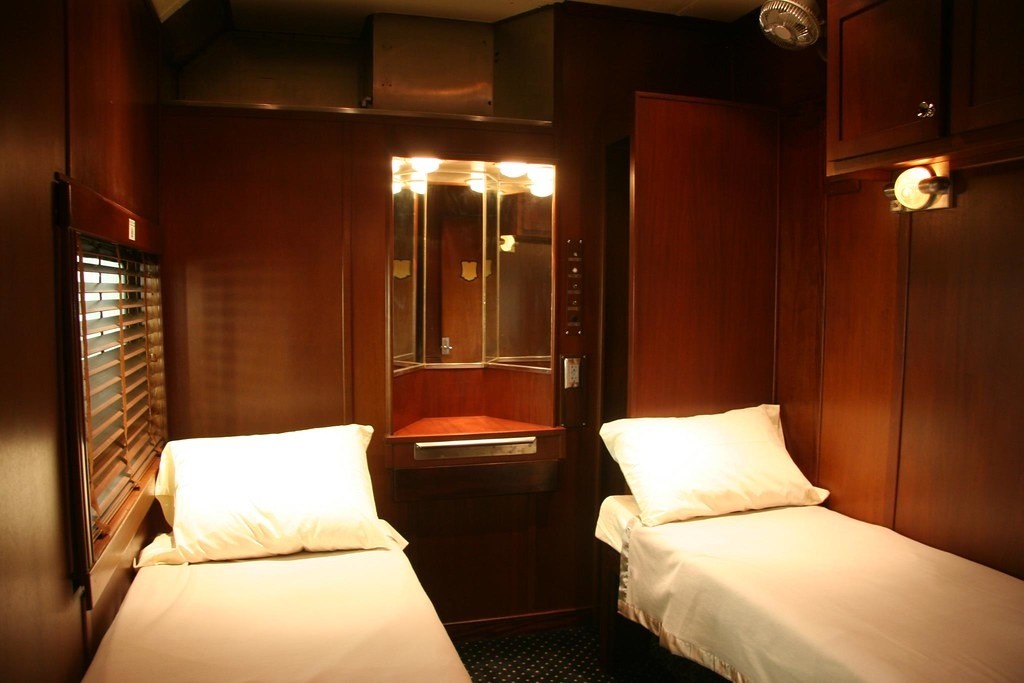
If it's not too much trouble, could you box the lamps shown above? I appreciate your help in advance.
[884,160,953,214]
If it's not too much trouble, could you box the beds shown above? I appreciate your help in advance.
[77,549,475,683]
[594,494,1024,683]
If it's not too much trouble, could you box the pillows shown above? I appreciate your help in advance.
[599,403,832,527]
[133,426,412,572]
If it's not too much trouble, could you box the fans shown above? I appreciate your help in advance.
[759,0,827,62]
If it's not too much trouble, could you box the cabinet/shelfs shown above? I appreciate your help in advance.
[826,0,1024,186]
[591,87,782,672]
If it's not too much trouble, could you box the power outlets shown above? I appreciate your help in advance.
[564,357,581,389]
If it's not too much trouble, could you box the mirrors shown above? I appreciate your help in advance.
[387,154,554,379]
